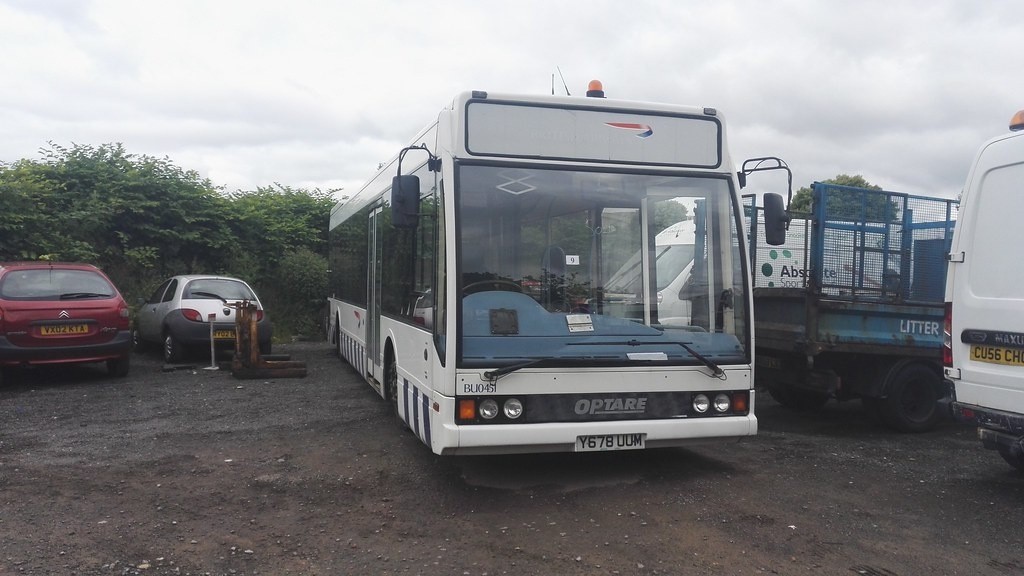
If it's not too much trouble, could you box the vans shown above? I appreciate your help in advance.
[944,108,1024,474]
[594,216,946,332]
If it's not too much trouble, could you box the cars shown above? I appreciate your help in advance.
[129,276,273,364]
[0,261,131,378]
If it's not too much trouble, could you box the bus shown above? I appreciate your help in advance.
[324,66,792,455]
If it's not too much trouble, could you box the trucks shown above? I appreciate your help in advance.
[688,180,962,435]
